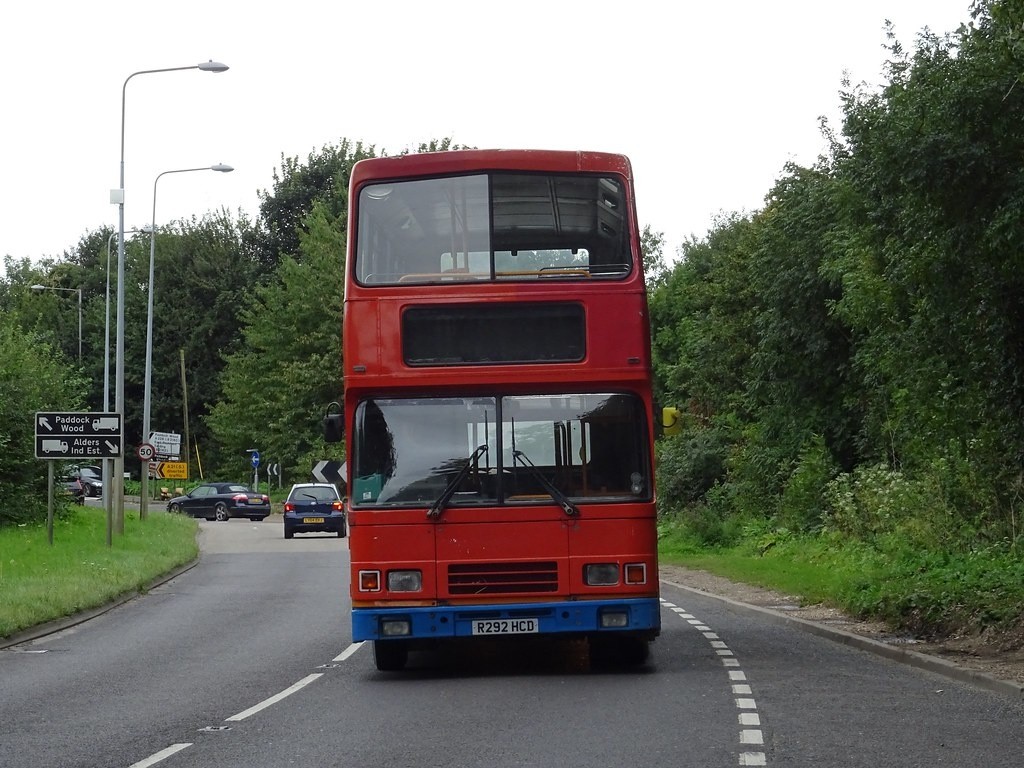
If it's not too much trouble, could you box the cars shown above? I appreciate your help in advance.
[165,483,272,522]
[281,482,349,539]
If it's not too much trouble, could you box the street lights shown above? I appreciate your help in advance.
[31,280,85,370]
[140,164,236,517]
[96,227,154,508]
[113,58,232,530]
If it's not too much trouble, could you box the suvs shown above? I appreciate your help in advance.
[71,465,102,497]
[54,476,84,505]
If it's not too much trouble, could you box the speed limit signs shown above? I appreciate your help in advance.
[138,443,156,460]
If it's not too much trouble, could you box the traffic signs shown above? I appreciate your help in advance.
[35,434,123,459]
[157,462,187,479]
[34,411,122,435]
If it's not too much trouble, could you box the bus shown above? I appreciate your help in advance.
[323,149,681,679]
